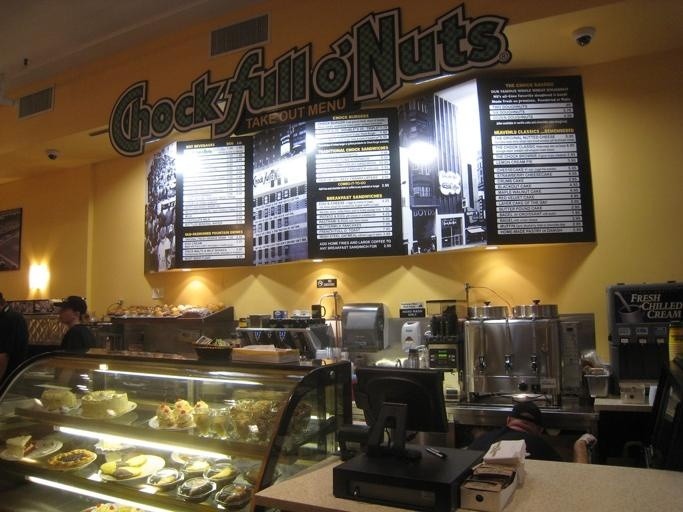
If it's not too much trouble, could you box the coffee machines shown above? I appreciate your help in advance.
[607,280,683,396]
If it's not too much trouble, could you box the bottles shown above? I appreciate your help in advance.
[668,321,682,362]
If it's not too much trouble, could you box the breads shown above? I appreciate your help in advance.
[100,455,147,479]
[47,448,93,468]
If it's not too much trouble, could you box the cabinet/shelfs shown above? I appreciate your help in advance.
[1,347,354,512]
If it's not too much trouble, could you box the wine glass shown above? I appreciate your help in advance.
[192,408,230,439]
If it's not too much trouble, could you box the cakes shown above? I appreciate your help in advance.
[156,395,210,428]
[6,434,36,458]
[41,387,77,411]
[81,389,129,417]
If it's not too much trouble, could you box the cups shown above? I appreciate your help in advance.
[340,347,349,361]
[619,304,646,324]
[311,304,326,318]
[408,349,419,369]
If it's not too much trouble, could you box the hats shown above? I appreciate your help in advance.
[53,295,87,314]
[509,402,545,426]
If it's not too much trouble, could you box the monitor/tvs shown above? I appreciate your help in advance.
[354,364,450,465]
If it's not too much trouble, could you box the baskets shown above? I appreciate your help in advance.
[192,342,233,359]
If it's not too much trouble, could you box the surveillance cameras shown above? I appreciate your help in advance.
[573,27,595,48]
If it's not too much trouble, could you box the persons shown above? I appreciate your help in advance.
[0,292,30,380]
[465,401,598,465]
[54,295,96,386]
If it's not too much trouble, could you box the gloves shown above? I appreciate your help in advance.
[578,433,599,449]
